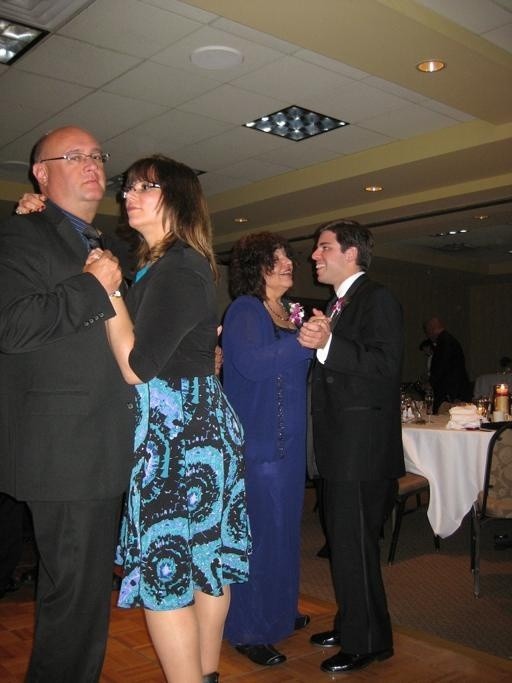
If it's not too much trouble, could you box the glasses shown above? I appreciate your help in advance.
[39,151,112,164]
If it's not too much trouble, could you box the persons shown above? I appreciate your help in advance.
[498,356,512,374]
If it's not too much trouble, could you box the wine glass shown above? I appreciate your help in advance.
[402,388,435,425]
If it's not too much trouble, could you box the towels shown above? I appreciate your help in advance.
[446,403,488,430]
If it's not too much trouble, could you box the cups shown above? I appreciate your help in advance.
[476,397,492,421]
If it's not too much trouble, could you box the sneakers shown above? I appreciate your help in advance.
[494,532,512,549]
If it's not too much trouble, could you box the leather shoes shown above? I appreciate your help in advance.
[232,638,288,667]
[293,613,311,630]
[0,568,39,599]
[309,630,341,648]
[318,639,395,674]
[111,575,123,591]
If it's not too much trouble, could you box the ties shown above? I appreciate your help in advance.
[120,179,161,199]
[82,223,100,249]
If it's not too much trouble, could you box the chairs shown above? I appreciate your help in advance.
[386,468,444,568]
[466,418,512,602]
